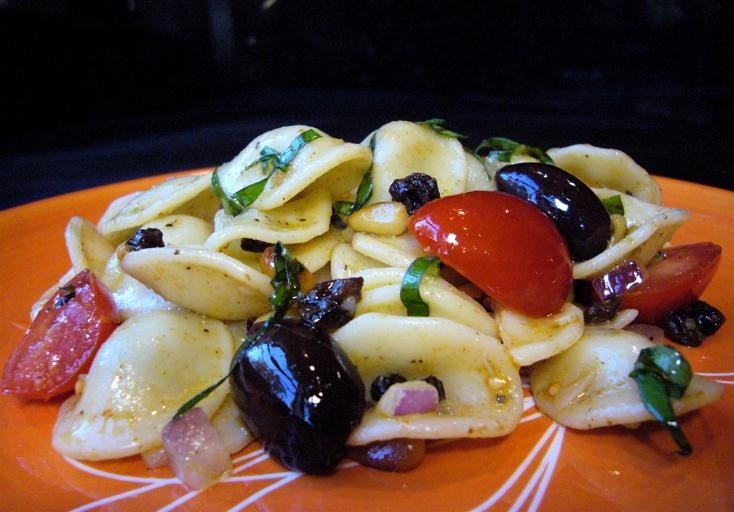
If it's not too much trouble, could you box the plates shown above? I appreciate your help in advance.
[1,164,734,511]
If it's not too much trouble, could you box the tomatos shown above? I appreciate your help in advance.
[3,270,120,401]
[409,189,575,315]
[597,242,724,324]
